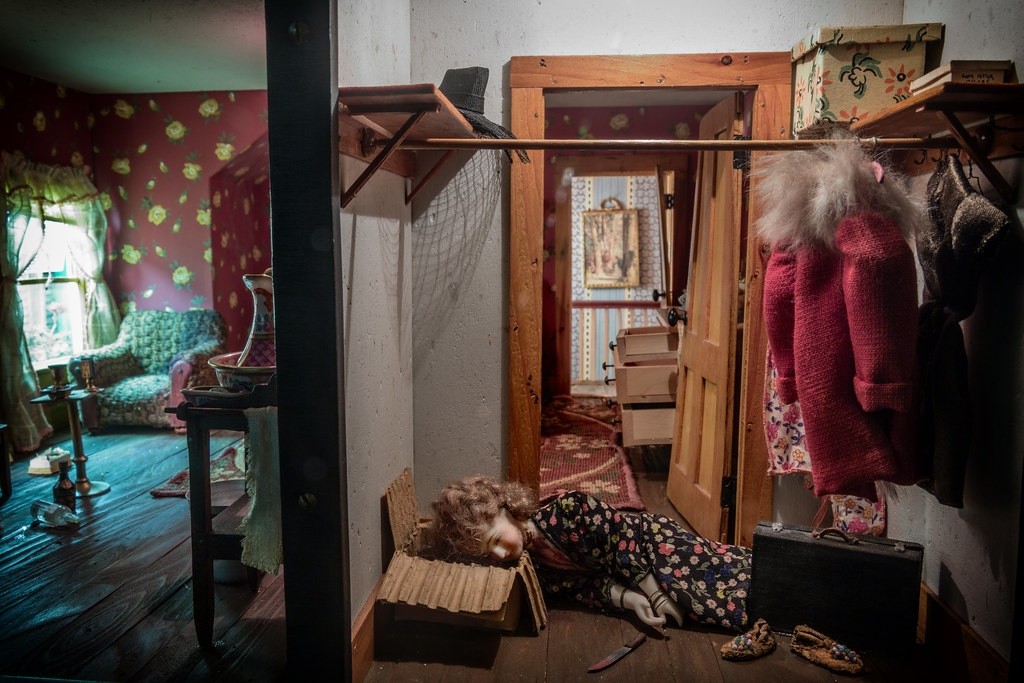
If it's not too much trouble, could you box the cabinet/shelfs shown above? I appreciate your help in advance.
[163,401,278,649]
[602,305,681,447]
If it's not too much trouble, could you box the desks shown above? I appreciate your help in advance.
[29,387,109,497]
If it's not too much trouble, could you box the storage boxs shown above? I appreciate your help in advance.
[909,60,1010,96]
[375,468,549,632]
[789,22,941,137]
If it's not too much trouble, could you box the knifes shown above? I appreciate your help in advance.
[588,632,646,670]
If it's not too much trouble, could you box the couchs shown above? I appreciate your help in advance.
[66,310,227,433]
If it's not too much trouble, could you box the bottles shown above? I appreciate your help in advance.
[53,458,75,515]
[236,268,275,367]
[30,499,79,527]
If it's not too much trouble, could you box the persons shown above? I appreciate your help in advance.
[432,474,754,638]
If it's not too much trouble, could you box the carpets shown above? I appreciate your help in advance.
[540,395,643,511]
[150,438,245,496]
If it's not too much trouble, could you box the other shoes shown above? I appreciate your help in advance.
[720,618,777,660]
[790,625,864,674]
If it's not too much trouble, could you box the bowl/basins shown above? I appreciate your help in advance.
[208,351,276,387]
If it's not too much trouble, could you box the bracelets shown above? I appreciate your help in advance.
[647,586,672,614]
[619,587,632,612]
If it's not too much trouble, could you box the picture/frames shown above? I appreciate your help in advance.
[583,198,642,290]
